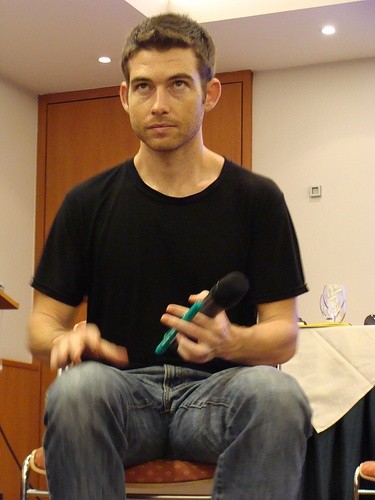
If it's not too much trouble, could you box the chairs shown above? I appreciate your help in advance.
[20,447,217,500]
[353,461,375,500]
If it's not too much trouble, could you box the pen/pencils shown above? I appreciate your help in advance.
[155,301,202,355]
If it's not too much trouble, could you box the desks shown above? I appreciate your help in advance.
[288,324,375,500]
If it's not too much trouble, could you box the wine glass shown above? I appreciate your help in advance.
[320,278,347,323]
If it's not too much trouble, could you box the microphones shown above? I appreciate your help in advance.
[198,272,249,318]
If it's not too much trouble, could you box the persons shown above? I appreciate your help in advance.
[25,12,310,500]
